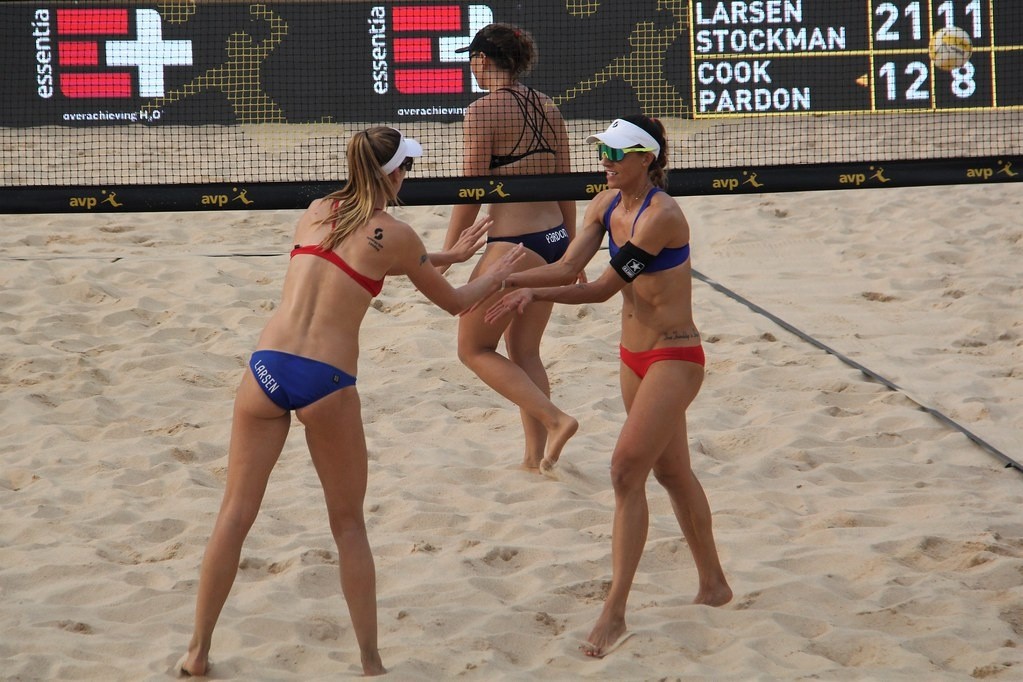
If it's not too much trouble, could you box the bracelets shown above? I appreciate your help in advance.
[498,280,505,292]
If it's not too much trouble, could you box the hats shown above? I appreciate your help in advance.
[586,119,659,160]
[373,127,423,178]
[455,33,513,70]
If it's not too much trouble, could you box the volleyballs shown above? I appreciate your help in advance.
[929,26,973,71]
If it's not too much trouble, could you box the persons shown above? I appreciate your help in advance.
[458,115,734,660]
[414,23,588,470]
[182,126,525,677]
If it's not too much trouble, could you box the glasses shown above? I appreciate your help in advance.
[469,54,480,61]
[595,143,657,162]
[399,157,413,171]
